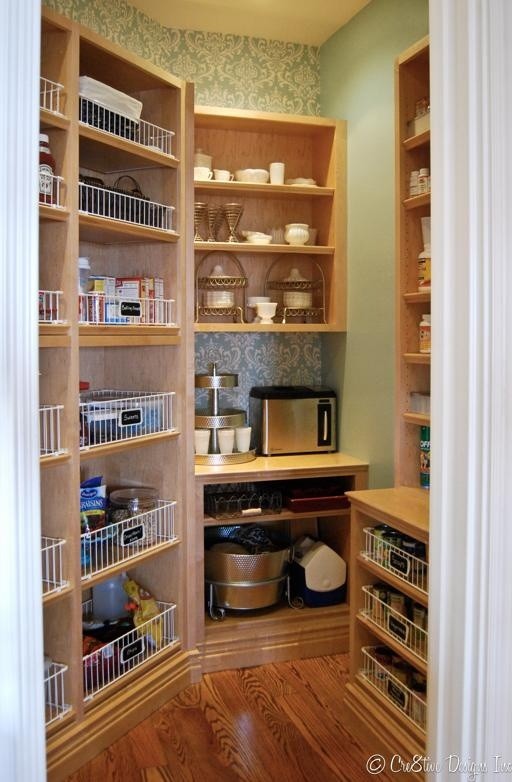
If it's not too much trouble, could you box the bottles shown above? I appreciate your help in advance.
[409,166,431,355]
[420,425,429,487]
[79,257,91,293]
[40,134,56,203]
[92,572,130,619]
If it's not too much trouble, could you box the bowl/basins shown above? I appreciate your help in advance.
[237,169,269,183]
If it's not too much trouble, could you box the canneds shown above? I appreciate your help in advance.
[76,293,89,324]
[87,291,106,324]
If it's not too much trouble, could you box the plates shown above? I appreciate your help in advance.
[202,291,235,306]
[283,292,312,307]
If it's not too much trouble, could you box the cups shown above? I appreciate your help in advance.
[214,169,235,180]
[194,426,253,454]
[269,163,285,185]
[194,167,213,179]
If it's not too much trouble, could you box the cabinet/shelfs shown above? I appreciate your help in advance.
[41,6,193,775]
[342,485,429,781]
[191,104,347,334]
[394,33,429,496]
[194,452,368,673]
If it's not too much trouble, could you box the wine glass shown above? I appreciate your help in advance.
[247,296,278,324]
